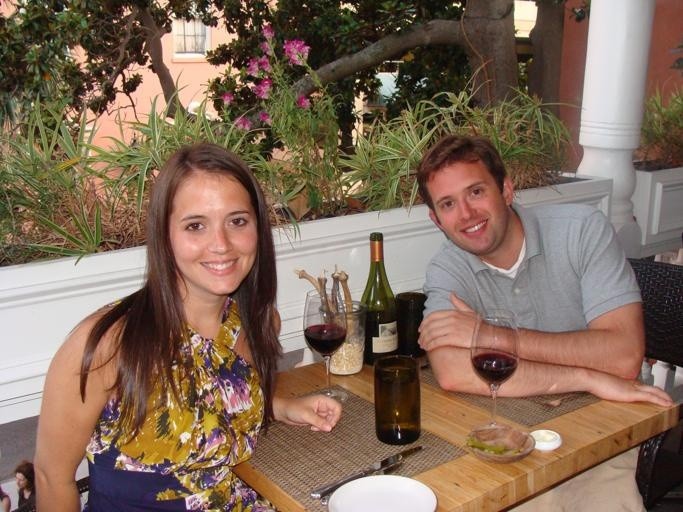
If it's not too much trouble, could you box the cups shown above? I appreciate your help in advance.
[319,301,364,376]
[374,353,422,445]
[395,292,430,357]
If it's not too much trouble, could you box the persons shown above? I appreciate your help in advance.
[32,142,342,512]
[0,463,36,512]
[416,135,683,407]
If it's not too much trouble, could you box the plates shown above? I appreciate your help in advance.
[328,474,437,512]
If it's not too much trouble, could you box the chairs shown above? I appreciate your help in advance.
[626,257,683,512]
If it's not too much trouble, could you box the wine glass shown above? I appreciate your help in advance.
[302,288,348,404]
[471,307,521,433]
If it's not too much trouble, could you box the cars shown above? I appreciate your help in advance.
[363,61,428,108]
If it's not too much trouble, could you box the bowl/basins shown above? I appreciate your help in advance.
[467,429,536,464]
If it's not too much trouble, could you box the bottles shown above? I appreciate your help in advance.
[359,233,399,363]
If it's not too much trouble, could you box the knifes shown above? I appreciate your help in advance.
[313,445,428,508]
